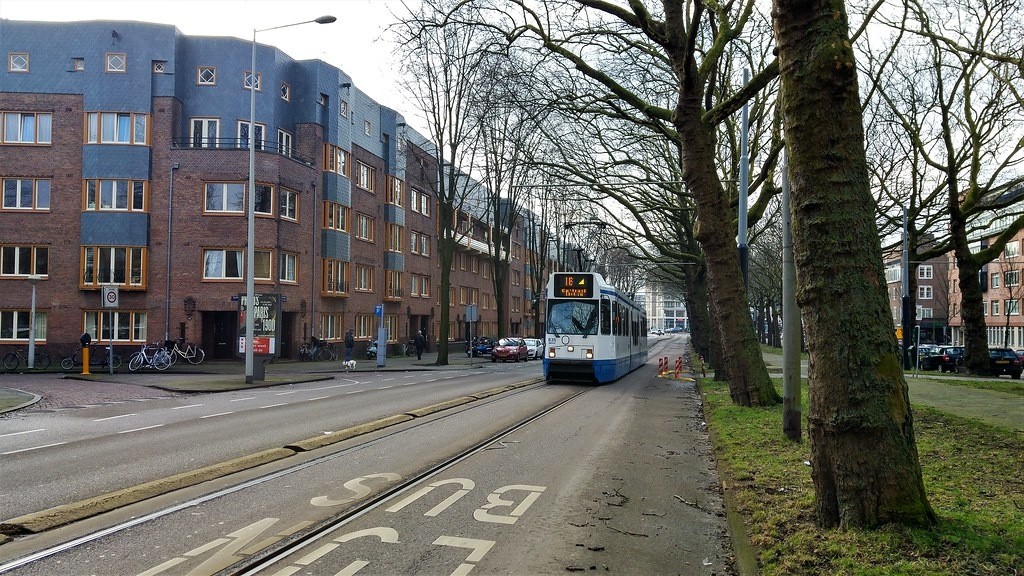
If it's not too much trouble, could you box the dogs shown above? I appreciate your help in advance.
[342,359,356,369]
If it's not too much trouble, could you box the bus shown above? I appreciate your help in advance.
[542,221,648,385]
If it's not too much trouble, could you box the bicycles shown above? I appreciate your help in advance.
[297,341,339,362]
[128,338,206,371]
[60,346,123,370]
[3,345,51,371]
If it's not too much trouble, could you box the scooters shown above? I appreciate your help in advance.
[366,340,378,360]
[465,337,478,357]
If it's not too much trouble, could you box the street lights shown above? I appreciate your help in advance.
[245,16,341,383]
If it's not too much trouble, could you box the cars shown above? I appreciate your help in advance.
[918,344,1024,380]
[524,339,544,360]
[651,326,690,335]
[480,337,488,345]
[492,337,529,362]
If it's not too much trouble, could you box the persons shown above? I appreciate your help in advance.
[344,329,355,362]
[414,331,426,360]
